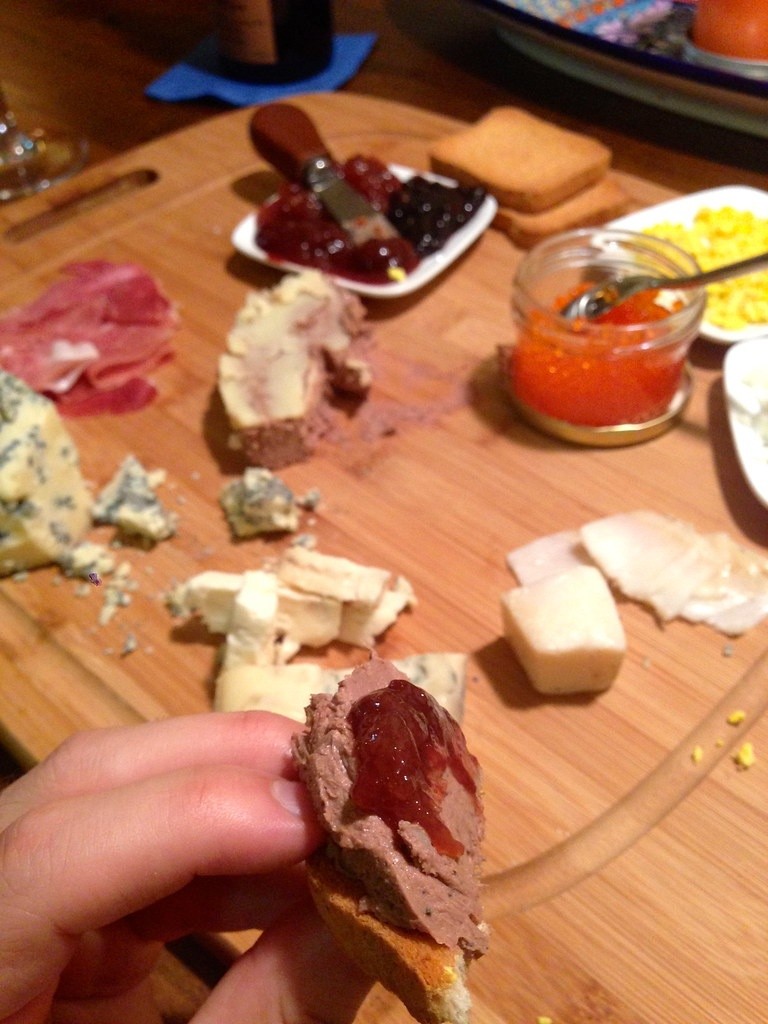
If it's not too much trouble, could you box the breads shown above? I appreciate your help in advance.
[428,105,630,250]
[216,270,380,470]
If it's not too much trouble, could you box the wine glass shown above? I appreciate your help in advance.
[0,84,90,201]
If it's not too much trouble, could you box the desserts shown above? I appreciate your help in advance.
[282,654,490,1024]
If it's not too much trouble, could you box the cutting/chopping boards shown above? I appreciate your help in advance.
[0,91,768,1024]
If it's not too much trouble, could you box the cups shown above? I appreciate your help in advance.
[510,229,706,444]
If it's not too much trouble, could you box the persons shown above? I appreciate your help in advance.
[2,710,376,1024]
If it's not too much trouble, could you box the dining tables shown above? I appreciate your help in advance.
[0,1,767,1024]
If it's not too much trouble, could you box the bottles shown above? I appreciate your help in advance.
[215,0,334,86]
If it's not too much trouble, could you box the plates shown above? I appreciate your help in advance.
[589,182,768,345]
[229,154,498,300]
[721,338,768,510]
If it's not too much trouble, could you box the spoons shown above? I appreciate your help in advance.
[560,244,768,322]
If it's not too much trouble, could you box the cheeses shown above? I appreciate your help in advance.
[0,365,303,585]
[173,544,469,728]
[498,560,626,696]
